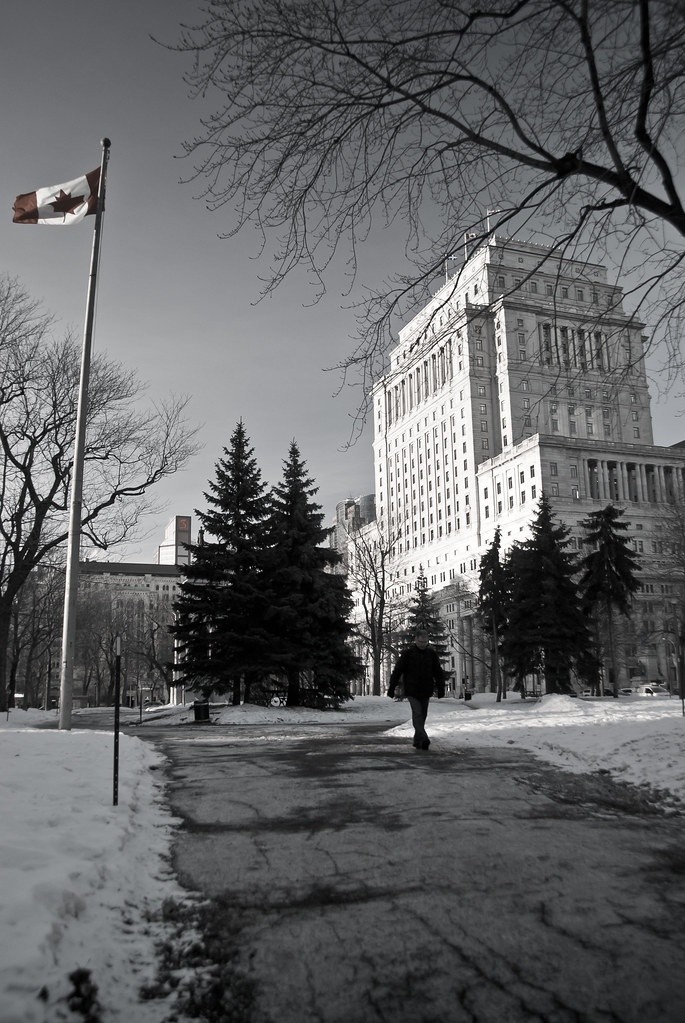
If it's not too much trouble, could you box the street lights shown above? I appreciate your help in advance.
[470,592,506,699]
[662,638,679,687]
[94,683,97,707]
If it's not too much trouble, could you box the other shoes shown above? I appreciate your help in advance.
[413,743,421,749]
[422,739,430,750]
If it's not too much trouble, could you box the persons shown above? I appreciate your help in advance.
[386,630,445,751]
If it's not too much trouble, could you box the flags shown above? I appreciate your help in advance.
[12,166,105,226]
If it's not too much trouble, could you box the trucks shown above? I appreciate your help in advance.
[39,699,80,710]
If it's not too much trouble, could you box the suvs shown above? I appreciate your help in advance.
[133,702,164,709]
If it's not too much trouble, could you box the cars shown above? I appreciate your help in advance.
[568,682,679,698]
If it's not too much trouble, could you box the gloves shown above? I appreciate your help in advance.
[387,688,395,698]
[438,689,445,698]
